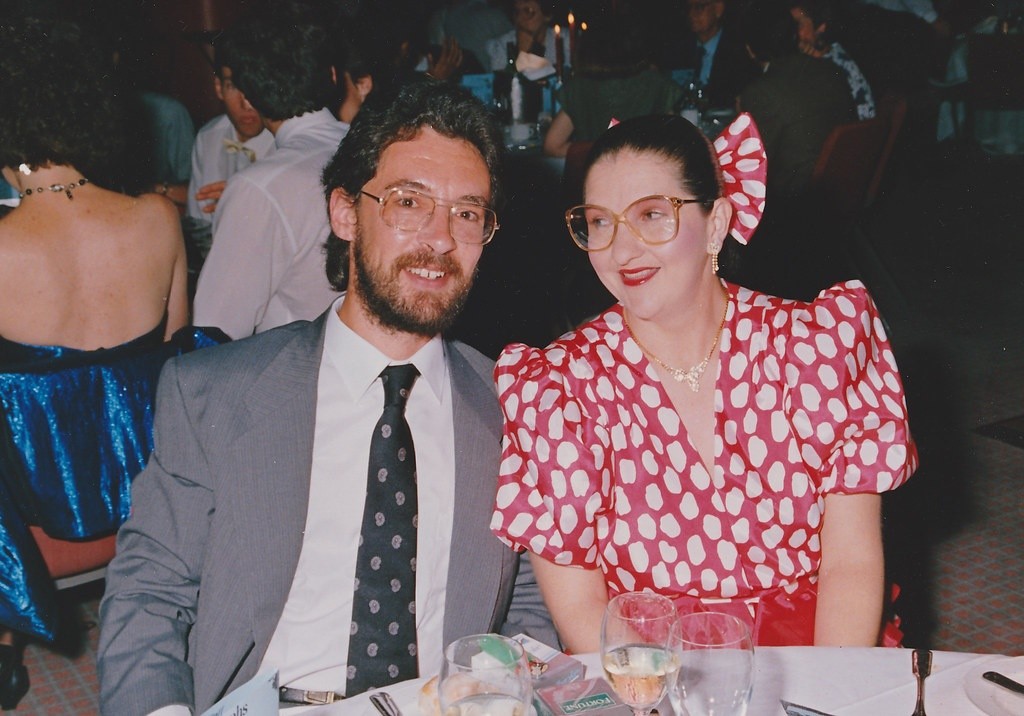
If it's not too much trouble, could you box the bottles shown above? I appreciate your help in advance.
[679,83,721,140]
[503,41,517,74]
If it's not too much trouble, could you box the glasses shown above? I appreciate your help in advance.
[357,187,499,245]
[684,0,718,13]
[565,193,713,251]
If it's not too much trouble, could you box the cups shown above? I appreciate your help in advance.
[437,633,533,715]
[510,125,534,140]
[664,612,756,716]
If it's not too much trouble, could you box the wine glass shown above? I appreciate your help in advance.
[601,590,683,715]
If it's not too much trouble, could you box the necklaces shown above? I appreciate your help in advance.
[20,177,90,201]
[616,287,728,393]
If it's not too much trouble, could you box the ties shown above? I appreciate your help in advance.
[344,364,422,701]
[692,45,706,80]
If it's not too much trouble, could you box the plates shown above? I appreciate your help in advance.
[961,655,1024,716]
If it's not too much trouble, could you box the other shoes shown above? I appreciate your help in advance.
[0,664,30,710]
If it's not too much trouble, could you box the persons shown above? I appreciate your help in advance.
[112,0,876,341]
[0,17,190,710]
[488,115,920,653]
[99,75,563,716]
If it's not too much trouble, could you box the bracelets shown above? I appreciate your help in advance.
[160,182,169,195]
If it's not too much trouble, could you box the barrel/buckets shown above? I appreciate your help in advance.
[493,69,543,126]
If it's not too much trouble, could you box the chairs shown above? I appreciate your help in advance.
[751,100,911,295]
[1,326,226,704]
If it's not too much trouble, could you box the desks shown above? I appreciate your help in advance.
[280,647,1023,715]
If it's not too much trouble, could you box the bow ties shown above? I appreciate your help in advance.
[224,137,256,163]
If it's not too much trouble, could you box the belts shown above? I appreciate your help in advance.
[278,684,346,704]
